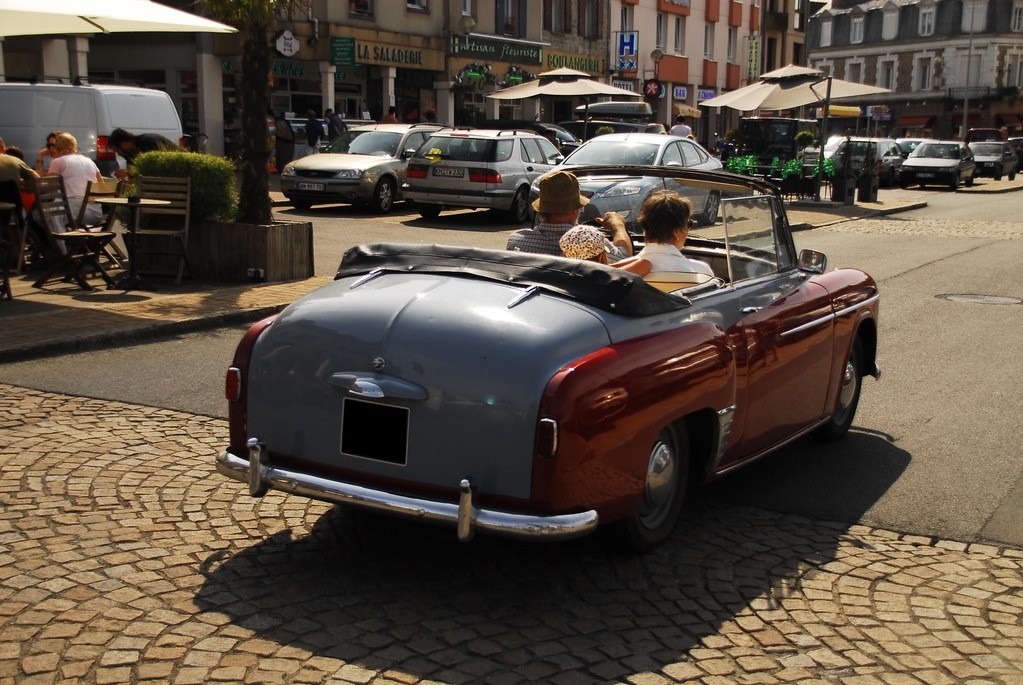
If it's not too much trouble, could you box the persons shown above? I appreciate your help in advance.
[42,132,104,227]
[304,110,325,155]
[382,106,398,122]
[0,137,41,222]
[669,114,691,138]
[108,128,186,180]
[558,225,651,277]
[506,171,633,265]
[5,146,24,161]
[617,189,715,293]
[324,109,347,142]
[35,133,63,177]
[275,115,294,173]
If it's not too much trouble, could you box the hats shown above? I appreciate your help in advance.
[533,171,591,213]
[560,225,605,260]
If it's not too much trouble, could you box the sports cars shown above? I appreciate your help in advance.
[213,164,878,548]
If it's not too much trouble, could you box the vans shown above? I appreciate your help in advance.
[965,128,1008,145]
[0,74,185,214]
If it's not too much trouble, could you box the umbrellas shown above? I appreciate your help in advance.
[484,67,642,124]
[698,64,892,202]
[0,0,239,37]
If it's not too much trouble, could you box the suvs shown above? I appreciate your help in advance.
[558,103,667,158]
[399,129,563,225]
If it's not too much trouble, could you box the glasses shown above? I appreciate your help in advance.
[683,219,693,232]
[579,206,584,212]
[46,142,56,148]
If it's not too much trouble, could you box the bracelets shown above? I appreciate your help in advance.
[37,160,43,164]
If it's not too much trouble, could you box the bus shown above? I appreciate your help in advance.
[739,118,816,169]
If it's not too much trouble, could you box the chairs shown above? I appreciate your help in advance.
[737,167,833,205]
[0,178,195,292]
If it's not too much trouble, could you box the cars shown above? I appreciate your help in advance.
[525,131,725,238]
[825,138,908,192]
[277,124,449,215]
[899,142,976,190]
[967,142,1020,182]
[1006,138,1023,168]
[477,119,581,158]
[894,137,937,158]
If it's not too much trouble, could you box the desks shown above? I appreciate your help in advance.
[94,194,171,288]
